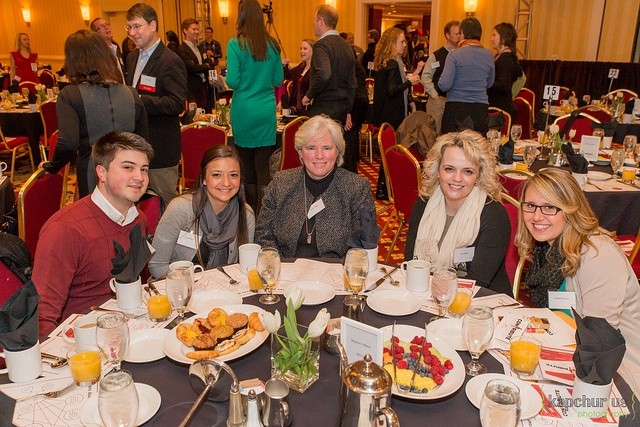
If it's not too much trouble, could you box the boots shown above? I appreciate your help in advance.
[376,182,390,200]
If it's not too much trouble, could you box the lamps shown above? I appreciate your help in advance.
[78,2,91,26]
[21,6,32,27]
[325,0,337,14]
[463,0,478,18]
[217,0,229,24]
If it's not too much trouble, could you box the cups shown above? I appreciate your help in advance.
[109,276,143,309]
[560,100,569,105]
[231,379,265,417]
[516,162,528,176]
[66,347,101,387]
[62,315,98,351]
[247,266,265,293]
[510,336,542,375]
[239,243,261,274]
[1,339,43,383]
[400,260,431,292]
[623,168,636,183]
[282,109,291,115]
[571,371,614,418]
[145,289,171,322]
[546,106,557,114]
[196,107,205,114]
[362,245,378,272]
[445,287,473,318]
[342,267,362,292]
[573,171,587,186]
[169,260,204,283]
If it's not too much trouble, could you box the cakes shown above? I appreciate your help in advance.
[192,318,212,335]
[208,307,228,326]
[186,350,218,361]
[232,329,256,345]
[214,339,240,355]
[226,313,249,329]
[176,322,198,348]
[210,326,234,343]
[249,312,265,332]
[193,335,217,350]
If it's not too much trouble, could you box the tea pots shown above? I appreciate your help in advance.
[335,341,400,427]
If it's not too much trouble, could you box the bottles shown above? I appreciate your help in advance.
[546,146,554,166]
[554,149,562,166]
[246,391,264,427]
[264,379,293,427]
[226,381,245,427]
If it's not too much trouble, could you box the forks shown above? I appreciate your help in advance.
[216,265,241,285]
[14,380,78,403]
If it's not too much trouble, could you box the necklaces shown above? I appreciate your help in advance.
[301,170,326,245]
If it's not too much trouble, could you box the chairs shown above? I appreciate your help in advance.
[377,121,398,205]
[1,122,41,178]
[488,105,511,148]
[500,191,532,299]
[512,96,532,139]
[517,87,537,117]
[549,82,569,108]
[276,113,315,172]
[178,122,230,194]
[18,162,73,272]
[383,145,423,263]
[39,98,64,159]
[549,113,598,139]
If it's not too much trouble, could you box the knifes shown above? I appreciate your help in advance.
[364,266,400,292]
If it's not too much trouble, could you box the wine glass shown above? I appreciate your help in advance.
[52,86,59,98]
[616,103,625,122]
[344,249,369,307]
[431,267,458,321]
[624,134,636,163]
[583,95,590,105]
[98,372,138,426]
[97,310,132,377]
[22,87,29,98]
[257,248,281,304]
[511,125,522,149]
[593,129,604,151]
[569,96,578,108]
[524,147,536,176]
[480,379,520,426]
[611,150,624,180]
[462,305,494,376]
[189,102,197,121]
[166,267,193,327]
[487,129,499,156]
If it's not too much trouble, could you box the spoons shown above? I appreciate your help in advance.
[41,357,68,369]
[381,266,400,286]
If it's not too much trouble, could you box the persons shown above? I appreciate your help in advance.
[301,5,359,174]
[346,32,364,69]
[148,144,256,281]
[175,19,216,115]
[373,27,420,200]
[165,29,179,53]
[225,0,287,211]
[405,128,513,299]
[488,22,523,137]
[509,167,640,401]
[420,21,463,133]
[121,2,189,213]
[362,29,380,77]
[411,28,428,60]
[36,28,149,199]
[339,32,358,59]
[90,17,127,85]
[253,112,379,263]
[410,61,425,80]
[120,36,133,65]
[198,25,224,74]
[337,32,358,61]
[10,33,43,96]
[281,38,316,115]
[30,131,156,344]
[437,17,496,140]
[127,39,137,52]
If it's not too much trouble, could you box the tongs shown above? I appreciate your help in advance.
[392,320,426,395]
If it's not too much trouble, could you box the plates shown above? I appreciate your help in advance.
[378,324,465,399]
[366,289,422,316]
[283,280,335,305]
[80,383,161,425]
[427,319,493,351]
[186,290,242,314]
[162,305,273,364]
[124,328,171,363]
[587,171,611,180]
[465,372,542,420]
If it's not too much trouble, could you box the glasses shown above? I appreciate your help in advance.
[96,22,109,30]
[520,202,562,215]
[125,22,150,29]
